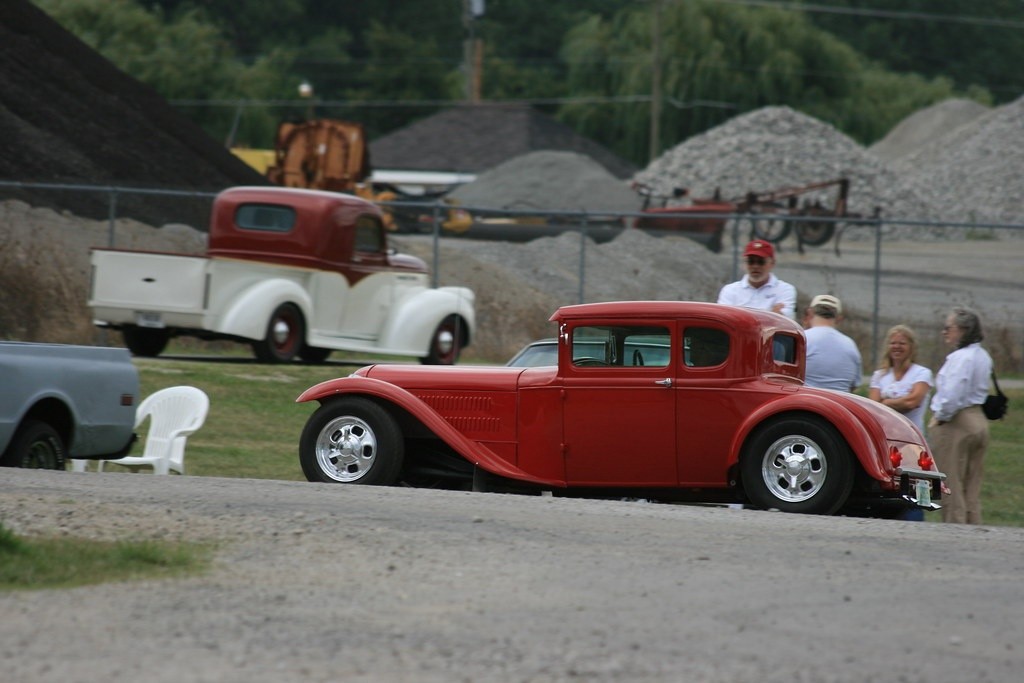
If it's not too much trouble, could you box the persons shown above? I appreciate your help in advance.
[868,324,936,522]
[718,239,797,363]
[929,308,994,525]
[804,295,863,393]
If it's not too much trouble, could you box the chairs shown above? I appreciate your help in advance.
[71,386,210,474]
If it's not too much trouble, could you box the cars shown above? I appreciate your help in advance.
[293,301,951,514]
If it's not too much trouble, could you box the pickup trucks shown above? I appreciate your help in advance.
[86,186,477,367]
[0,341,138,470]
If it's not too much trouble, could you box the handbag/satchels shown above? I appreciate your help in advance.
[981,395,1010,420]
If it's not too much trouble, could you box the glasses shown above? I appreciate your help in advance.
[944,325,956,330]
[745,257,770,266]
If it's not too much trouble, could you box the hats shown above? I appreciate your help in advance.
[809,295,843,313]
[741,239,775,259]
